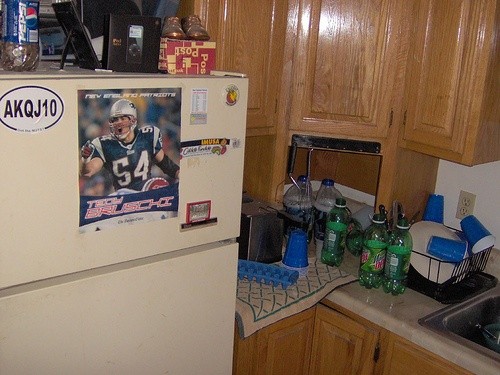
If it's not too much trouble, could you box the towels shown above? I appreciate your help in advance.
[236,258,359,340]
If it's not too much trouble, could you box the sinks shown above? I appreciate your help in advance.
[416,284,499,363]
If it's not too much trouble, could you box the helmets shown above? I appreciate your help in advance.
[108,99,137,141]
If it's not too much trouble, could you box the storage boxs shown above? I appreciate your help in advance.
[158,37,216,73]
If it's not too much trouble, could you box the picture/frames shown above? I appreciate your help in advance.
[51,0,101,72]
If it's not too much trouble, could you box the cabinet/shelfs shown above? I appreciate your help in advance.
[404,1,500,166]
[177,0,286,136]
[233,307,315,375]
[316,299,379,375]
[286,0,404,142]
[379,325,479,375]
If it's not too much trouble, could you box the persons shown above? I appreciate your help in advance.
[81,99,180,197]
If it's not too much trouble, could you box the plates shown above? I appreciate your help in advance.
[409,220,468,284]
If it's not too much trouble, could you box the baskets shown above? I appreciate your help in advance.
[408,220,494,289]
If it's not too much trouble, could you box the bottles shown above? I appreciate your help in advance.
[321,197,351,268]
[382,219,413,296]
[359,213,389,289]
[315,178,342,259]
[346,216,365,256]
[0,0,41,72]
[284,175,311,245]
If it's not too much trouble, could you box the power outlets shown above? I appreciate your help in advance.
[455,189,476,220]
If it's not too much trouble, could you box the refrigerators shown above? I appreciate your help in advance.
[0,62,249,375]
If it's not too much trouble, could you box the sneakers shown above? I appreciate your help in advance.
[162,16,186,39]
[181,15,209,40]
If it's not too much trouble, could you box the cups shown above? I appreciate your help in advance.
[425,235,469,266]
[280,231,310,278]
[422,193,444,224]
[460,215,496,254]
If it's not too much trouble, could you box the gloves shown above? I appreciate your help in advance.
[81,140,95,164]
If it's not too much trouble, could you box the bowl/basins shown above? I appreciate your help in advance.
[483,323,500,353]
